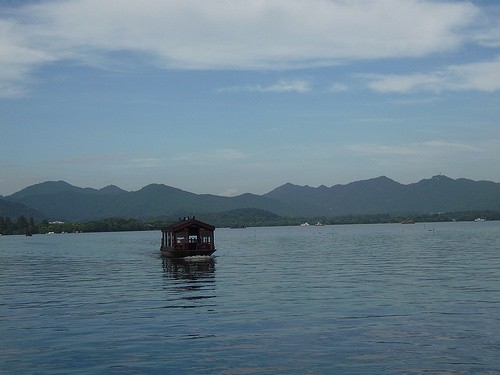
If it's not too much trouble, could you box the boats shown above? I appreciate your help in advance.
[160,215,217,258]
